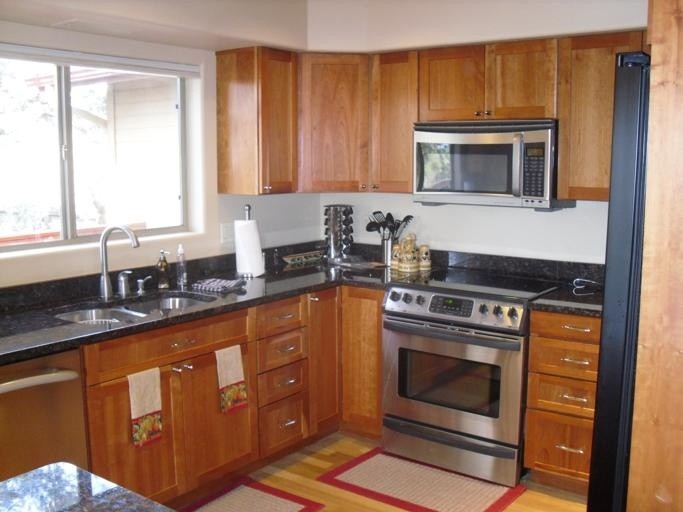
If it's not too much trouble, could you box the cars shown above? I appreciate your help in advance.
[380,239,399,267]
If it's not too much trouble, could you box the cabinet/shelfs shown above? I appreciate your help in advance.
[390,234,431,272]
[175,243,188,285]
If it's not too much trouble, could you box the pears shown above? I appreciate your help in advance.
[177,244,189,285]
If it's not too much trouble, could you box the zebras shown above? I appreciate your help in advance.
[378,313,524,489]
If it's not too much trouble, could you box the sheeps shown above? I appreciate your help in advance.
[99,224,140,298]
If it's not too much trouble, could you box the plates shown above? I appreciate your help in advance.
[301,284,343,445]
[215,45,298,194]
[81,310,256,498]
[522,310,602,499]
[555,30,645,201]
[416,38,560,121]
[341,285,385,439]
[287,52,419,194]
[248,295,309,471]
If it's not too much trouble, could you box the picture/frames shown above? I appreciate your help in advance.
[0,460,179,511]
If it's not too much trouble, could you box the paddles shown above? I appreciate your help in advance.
[182,476,325,510]
[316,446,526,510]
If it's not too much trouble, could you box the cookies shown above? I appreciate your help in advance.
[411,118,575,212]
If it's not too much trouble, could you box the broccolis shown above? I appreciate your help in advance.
[583,47,652,512]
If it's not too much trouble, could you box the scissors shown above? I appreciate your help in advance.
[373,211,386,231]
